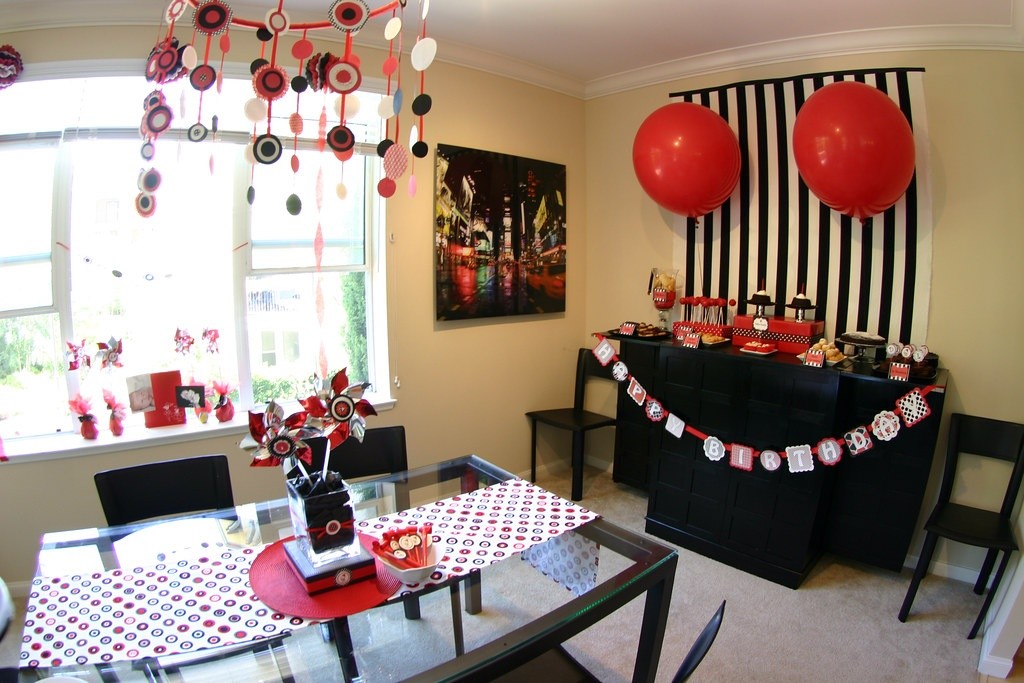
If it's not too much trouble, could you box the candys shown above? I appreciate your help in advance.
[680,296,736,327]
[888,342,928,368]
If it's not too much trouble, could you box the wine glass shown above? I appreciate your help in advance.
[651,268,679,331]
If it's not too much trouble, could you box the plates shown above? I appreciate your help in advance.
[701,339,731,346]
[740,348,778,356]
[608,329,673,339]
[796,353,847,366]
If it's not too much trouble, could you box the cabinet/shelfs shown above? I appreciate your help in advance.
[600,329,948,590]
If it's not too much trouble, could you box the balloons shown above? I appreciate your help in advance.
[792,81,916,223]
[632,102,741,222]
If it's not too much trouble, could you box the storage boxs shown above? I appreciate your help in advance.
[732,314,824,354]
[143,370,187,429]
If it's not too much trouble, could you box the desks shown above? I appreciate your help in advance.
[18,455,680,683]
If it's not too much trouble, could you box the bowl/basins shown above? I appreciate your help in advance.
[378,543,447,584]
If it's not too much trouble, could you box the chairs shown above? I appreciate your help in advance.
[94,454,295,683]
[286,425,422,644]
[490,601,726,683]
[525,349,621,502]
[897,412,1024,642]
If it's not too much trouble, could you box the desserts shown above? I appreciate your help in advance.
[790,294,811,306]
[752,290,770,302]
[756,343,775,352]
[810,338,844,360]
[745,341,760,351]
[842,332,886,345]
